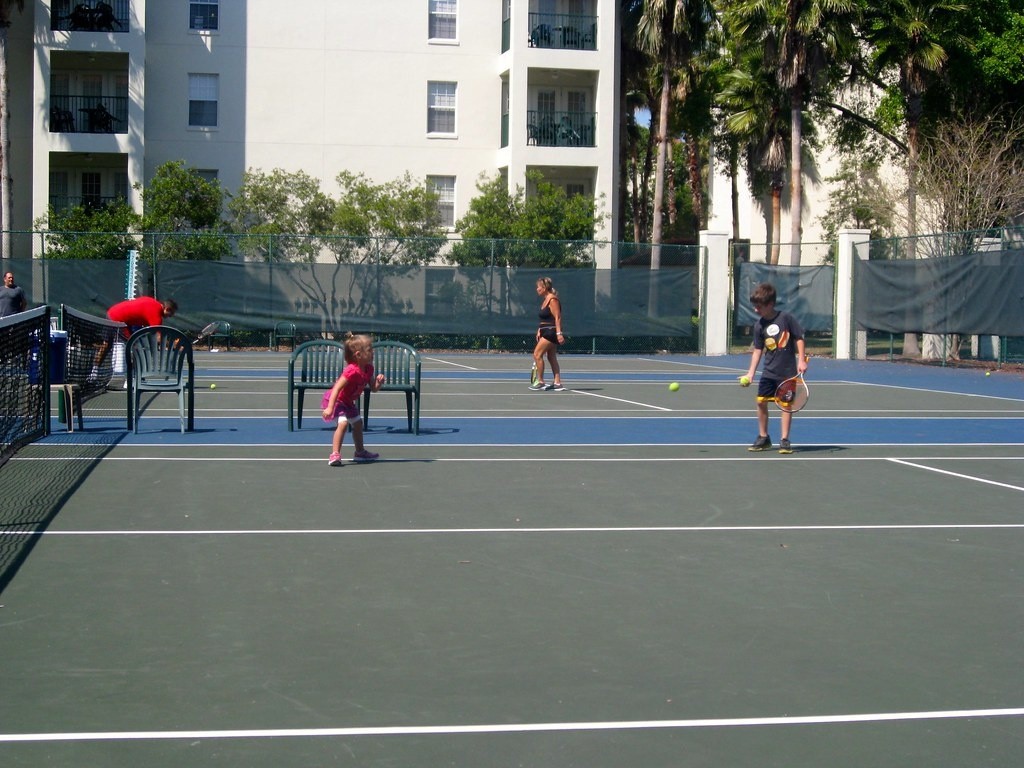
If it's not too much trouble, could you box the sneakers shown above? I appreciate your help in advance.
[528,379,545,390]
[545,384,566,391]
[355,450,379,461]
[779,438,793,454]
[329,452,341,466]
[748,435,771,450]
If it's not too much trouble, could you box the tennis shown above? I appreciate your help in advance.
[985,372,991,376]
[669,382,680,393]
[739,376,751,386]
[210,383,216,390]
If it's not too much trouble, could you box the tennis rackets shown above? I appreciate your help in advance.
[774,356,810,413]
[530,337,540,384]
[190,320,221,345]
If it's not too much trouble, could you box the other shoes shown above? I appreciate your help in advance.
[86,375,97,383]
[123,378,135,388]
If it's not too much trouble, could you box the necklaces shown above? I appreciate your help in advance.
[543,294,553,304]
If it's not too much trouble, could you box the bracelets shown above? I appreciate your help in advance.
[556,332,563,335]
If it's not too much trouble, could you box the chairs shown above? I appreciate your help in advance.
[527,116,591,147]
[208,320,231,351]
[288,340,351,432]
[64,0,123,32]
[529,23,597,50]
[125,325,195,435]
[50,103,122,133]
[356,341,421,435]
[274,321,296,352]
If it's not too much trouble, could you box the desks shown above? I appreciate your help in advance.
[553,26,577,48]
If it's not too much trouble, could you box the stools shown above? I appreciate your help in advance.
[32,384,84,434]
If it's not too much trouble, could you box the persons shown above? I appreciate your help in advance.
[85,296,178,389]
[320,331,387,466]
[0,273,27,317]
[737,283,808,454]
[527,277,567,391]
